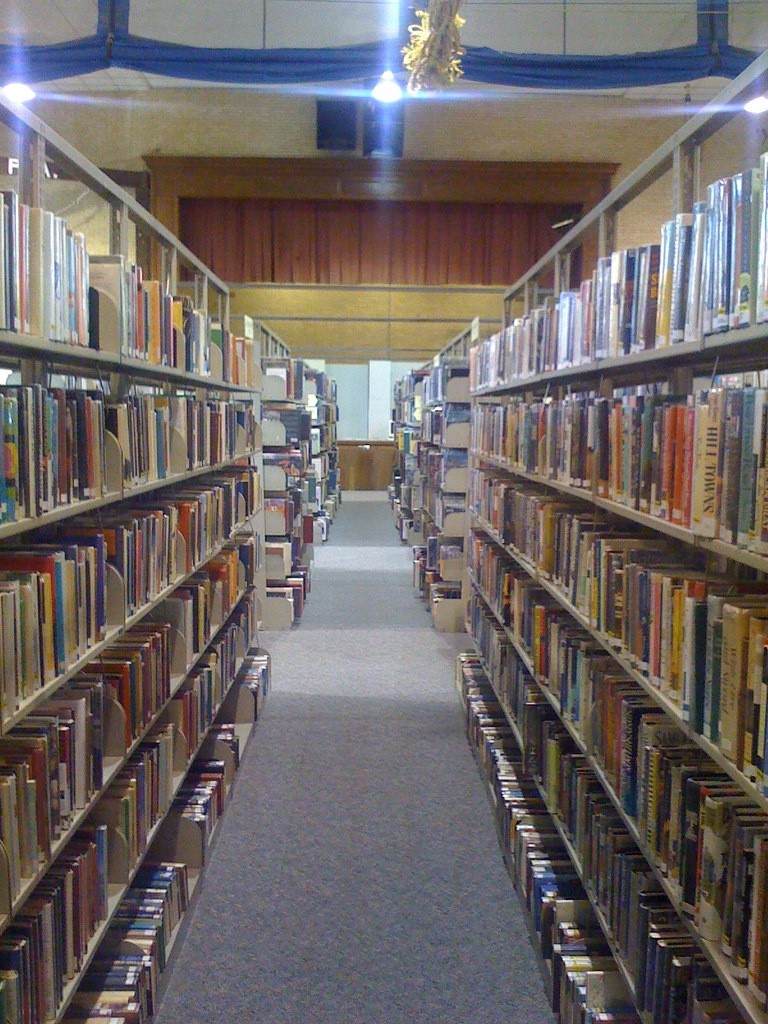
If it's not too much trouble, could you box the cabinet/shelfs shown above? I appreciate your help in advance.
[0,89,342,1024]
[389,49,768,1024]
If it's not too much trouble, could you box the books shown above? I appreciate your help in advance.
[0,189,341,1024]
[389,154,768,1024]
